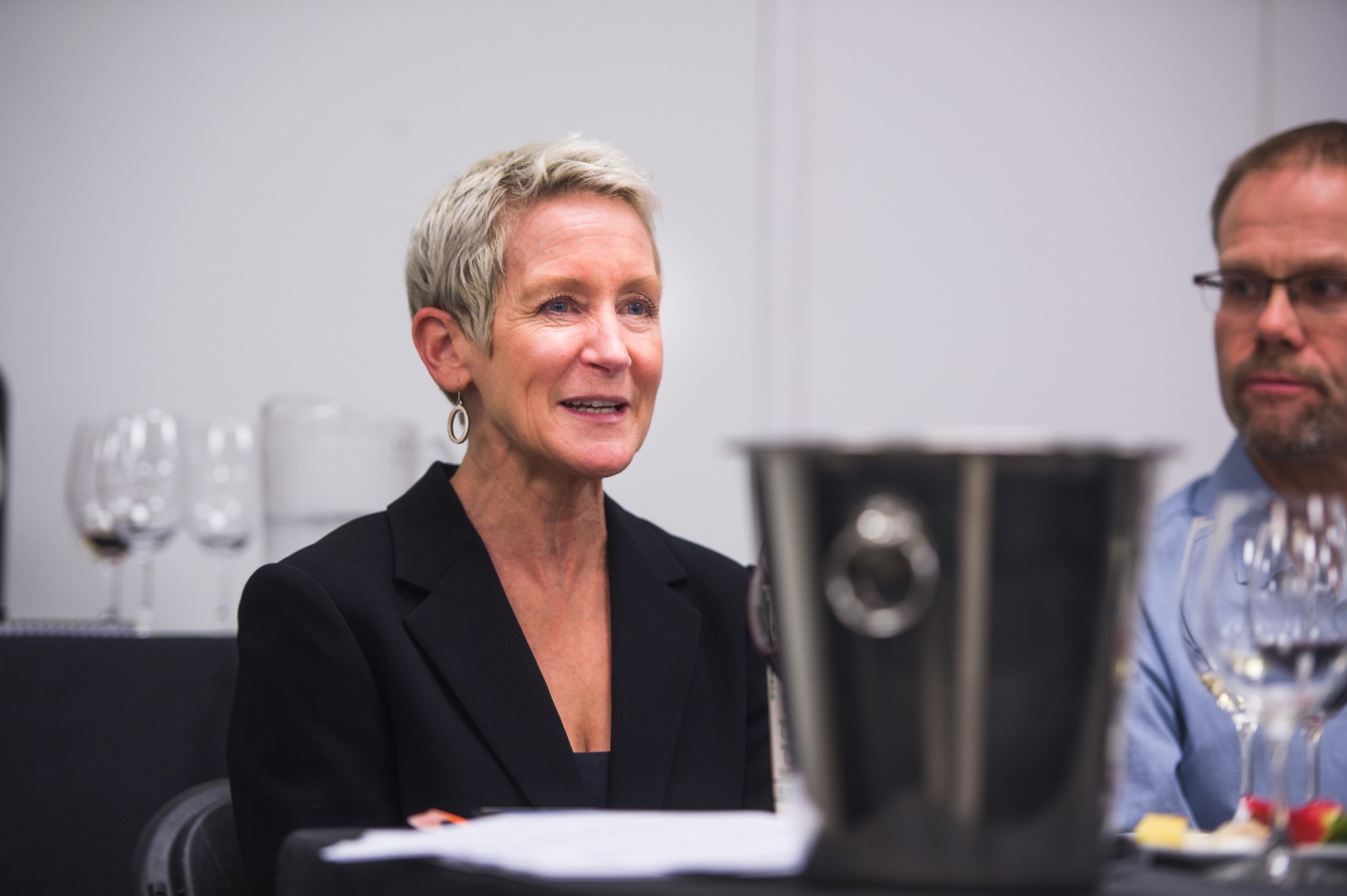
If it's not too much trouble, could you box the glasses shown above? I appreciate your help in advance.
[1195,270,1347,312]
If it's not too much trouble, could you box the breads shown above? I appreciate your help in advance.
[1133,813,1188,851]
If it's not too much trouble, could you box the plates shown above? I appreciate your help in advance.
[1116,829,1347,863]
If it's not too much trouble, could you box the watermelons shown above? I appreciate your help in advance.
[1246,795,1347,848]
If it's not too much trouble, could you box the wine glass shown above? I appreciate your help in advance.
[1173,488,1347,895]
[95,406,190,632]
[62,421,135,618]
[189,413,262,636]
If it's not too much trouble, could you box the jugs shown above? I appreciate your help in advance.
[259,398,422,559]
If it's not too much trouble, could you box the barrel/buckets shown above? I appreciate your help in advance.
[730,443,1173,823]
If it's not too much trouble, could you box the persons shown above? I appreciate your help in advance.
[233,141,780,893]
[1099,117,1347,856]
